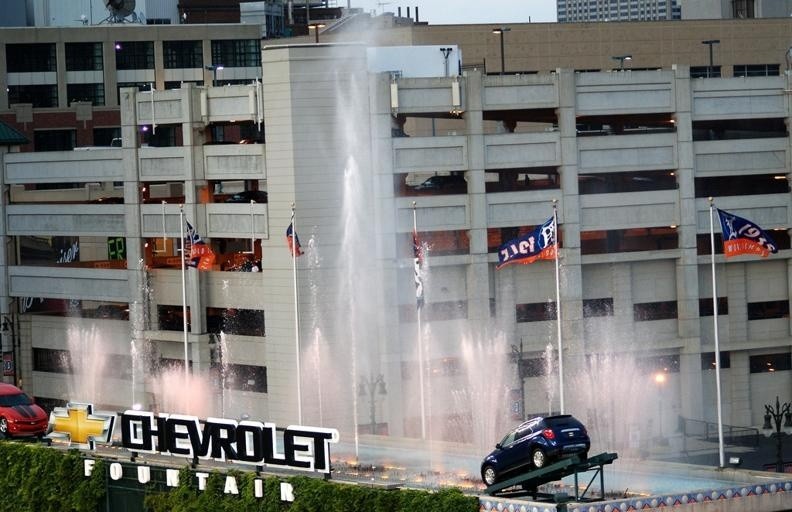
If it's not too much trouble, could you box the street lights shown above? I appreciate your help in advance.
[651,371,668,446]
[3,316,17,387]
[764,395,792,473]
[359,373,387,435]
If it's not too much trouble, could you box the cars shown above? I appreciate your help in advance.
[414,175,467,194]
[225,190,267,203]
[109,305,129,320]
[480,414,591,487]
[0,383,49,438]
[95,304,120,319]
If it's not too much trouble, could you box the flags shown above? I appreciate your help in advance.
[715,207,779,257]
[413,229,424,307]
[494,210,559,270]
[286,223,301,257]
[182,214,215,273]
